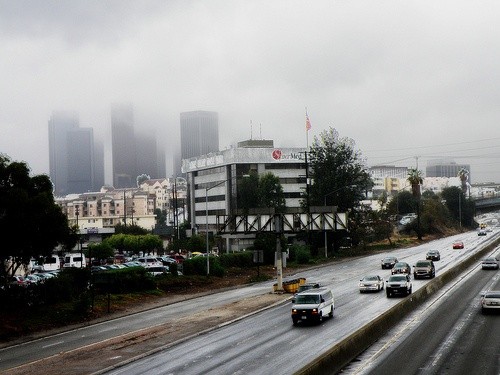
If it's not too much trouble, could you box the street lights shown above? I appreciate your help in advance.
[397,184,423,242]
[205,174,250,284]
[324,184,357,259]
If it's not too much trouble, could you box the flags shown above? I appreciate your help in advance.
[306,112,311,131]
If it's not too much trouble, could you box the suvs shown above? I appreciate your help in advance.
[412,259,435,280]
[386,274,412,298]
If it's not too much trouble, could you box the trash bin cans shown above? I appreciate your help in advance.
[273,277,306,293]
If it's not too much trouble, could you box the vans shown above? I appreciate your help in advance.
[63,253,87,268]
[28,254,60,274]
[291,287,335,327]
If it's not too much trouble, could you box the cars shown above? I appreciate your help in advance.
[359,274,384,294]
[381,257,398,270]
[92,251,220,279]
[453,241,464,249]
[292,283,319,303]
[481,291,500,313]
[426,250,440,261]
[482,257,499,270]
[478,222,492,236]
[391,262,411,275]
[0,271,60,289]
